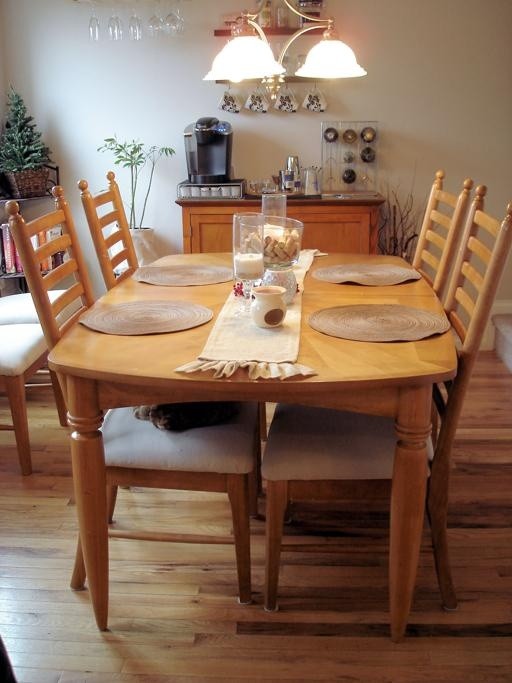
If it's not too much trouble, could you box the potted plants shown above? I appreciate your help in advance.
[98,133,175,270]
[0,82,55,197]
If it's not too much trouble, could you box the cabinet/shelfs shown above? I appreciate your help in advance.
[176,193,388,257]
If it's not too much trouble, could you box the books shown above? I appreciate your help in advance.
[2,223,61,274]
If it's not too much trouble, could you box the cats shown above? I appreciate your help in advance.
[133,401,244,432]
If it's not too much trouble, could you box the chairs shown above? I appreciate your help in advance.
[0,288,85,323]
[4,187,258,604]
[76,171,138,289]
[410,169,473,295]
[260,184,512,613]
[0,324,67,476]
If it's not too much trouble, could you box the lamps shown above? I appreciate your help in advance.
[201,0,369,82]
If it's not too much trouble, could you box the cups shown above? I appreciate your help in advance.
[252,285,287,328]
[280,169,295,192]
[217,85,329,115]
[262,194,287,216]
[180,187,242,198]
[263,269,296,305]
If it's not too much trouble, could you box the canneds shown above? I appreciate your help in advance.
[279,170,294,193]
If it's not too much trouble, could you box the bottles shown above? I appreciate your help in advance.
[304,169,318,196]
[287,156,299,174]
[257,0,289,29]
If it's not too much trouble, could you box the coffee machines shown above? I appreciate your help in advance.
[178,117,246,195]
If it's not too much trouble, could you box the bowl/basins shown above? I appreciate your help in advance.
[240,215,304,269]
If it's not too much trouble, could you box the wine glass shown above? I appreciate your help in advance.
[233,212,264,315]
[89,2,185,42]
